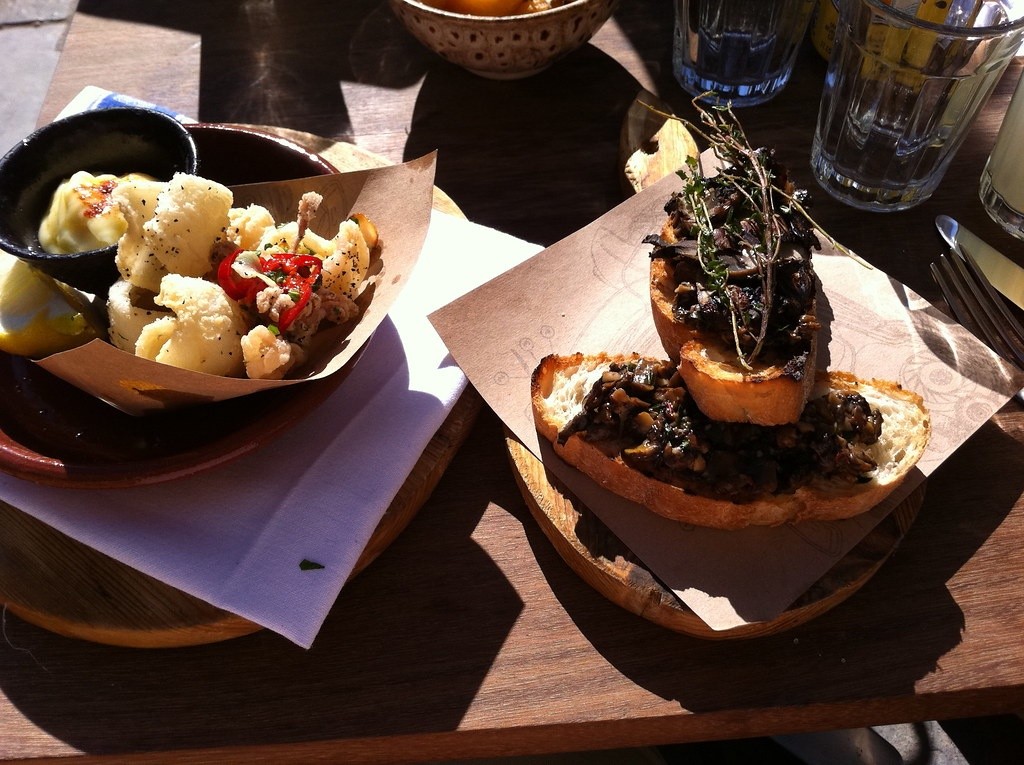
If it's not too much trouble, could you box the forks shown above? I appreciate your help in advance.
[929,242,1024,404]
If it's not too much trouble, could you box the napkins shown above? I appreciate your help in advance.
[0,208,546,649]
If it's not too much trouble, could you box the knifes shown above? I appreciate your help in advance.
[934,214,1024,315]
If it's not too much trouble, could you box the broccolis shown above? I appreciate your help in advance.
[86,172,379,380]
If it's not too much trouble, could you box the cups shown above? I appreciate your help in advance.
[812,1,1024,215]
[975,66,1024,246]
[666,1,827,108]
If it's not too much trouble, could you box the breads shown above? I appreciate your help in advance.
[531,175,931,528]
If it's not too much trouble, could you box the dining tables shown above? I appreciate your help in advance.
[0,0,1024,765]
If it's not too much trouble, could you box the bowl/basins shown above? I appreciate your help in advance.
[0,122,385,489]
[0,109,200,295]
[391,0,616,80]
